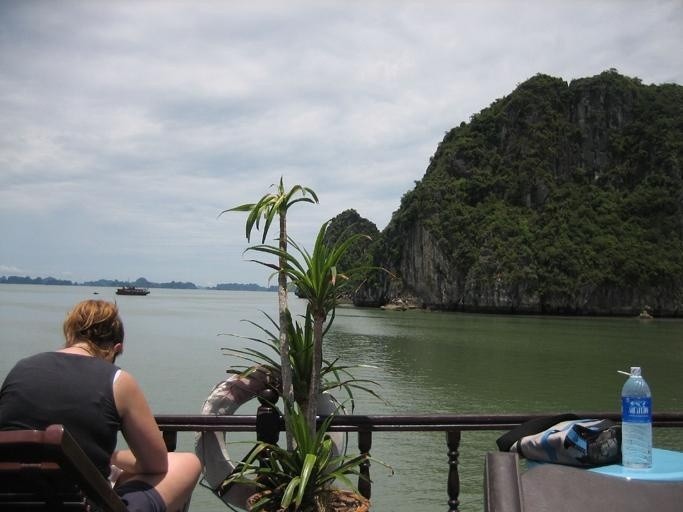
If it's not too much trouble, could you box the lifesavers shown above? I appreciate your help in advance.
[194,366,348,511]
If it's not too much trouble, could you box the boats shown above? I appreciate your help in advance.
[115,286,150,296]
[93,292,99,294]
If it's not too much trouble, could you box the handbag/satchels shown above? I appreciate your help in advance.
[496,413,623,469]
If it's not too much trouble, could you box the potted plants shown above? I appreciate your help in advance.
[213,171,396,512]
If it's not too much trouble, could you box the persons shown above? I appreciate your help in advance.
[0,298,204,512]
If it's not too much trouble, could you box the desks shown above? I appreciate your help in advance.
[481,444,683,511]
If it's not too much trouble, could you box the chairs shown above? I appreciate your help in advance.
[0,421,127,512]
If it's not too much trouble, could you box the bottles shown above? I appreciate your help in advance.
[620,366,653,471]
